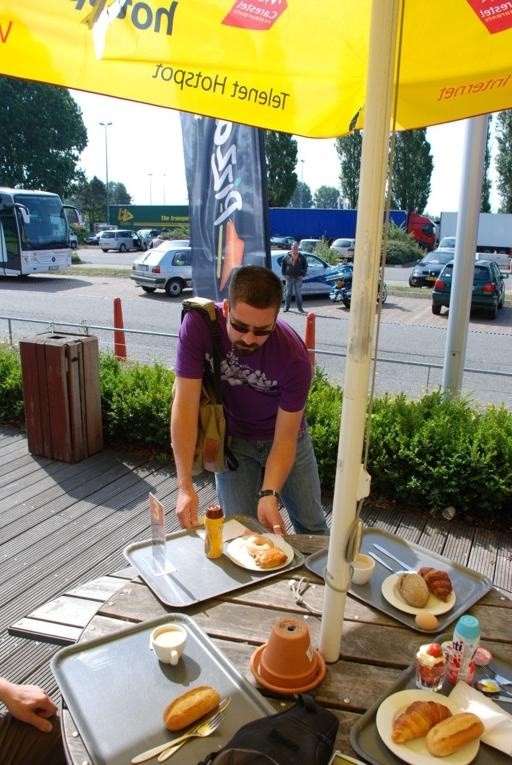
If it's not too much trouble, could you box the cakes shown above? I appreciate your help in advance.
[417,641,449,686]
[476,679,500,692]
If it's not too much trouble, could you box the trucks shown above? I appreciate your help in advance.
[433,210,512,257]
[268,206,439,254]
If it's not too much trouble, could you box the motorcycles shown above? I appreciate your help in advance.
[324,260,389,310]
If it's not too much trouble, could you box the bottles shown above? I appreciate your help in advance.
[448,615,480,686]
[204,504,224,559]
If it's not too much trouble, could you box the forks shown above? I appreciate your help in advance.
[482,666,512,687]
[131,696,231,763]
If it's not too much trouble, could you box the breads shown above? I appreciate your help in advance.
[162,682,221,732]
[391,701,486,757]
[247,536,289,569]
[397,567,453,607]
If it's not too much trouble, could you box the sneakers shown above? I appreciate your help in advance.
[282,306,305,314]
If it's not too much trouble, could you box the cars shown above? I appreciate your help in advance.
[128,232,356,305]
[408,235,507,320]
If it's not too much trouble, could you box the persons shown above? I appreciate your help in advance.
[280,241,307,313]
[0,676,69,765]
[169,266,330,540]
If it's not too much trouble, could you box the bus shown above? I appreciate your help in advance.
[0,186,84,281]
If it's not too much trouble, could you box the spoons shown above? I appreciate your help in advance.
[475,677,512,699]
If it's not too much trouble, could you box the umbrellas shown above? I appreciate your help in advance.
[0,0,512,664]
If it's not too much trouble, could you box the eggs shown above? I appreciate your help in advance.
[414,612,439,630]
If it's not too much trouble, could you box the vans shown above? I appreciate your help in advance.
[68,223,166,254]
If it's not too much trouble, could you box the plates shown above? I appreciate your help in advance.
[227,534,294,571]
[377,689,481,765]
[382,570,456,616]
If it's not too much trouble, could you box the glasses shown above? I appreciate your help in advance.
[228,306,277,337]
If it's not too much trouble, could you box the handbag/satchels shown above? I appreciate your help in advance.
[167,368,239,478]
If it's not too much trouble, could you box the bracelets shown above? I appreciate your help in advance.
[255,489,279,501]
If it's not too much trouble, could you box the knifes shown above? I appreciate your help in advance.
[369,543,414,575]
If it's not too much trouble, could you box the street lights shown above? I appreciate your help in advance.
[148,173,153,206]
[98,120,113,224]
[299,159,305,210]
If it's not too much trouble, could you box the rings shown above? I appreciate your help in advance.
[272,524,281,529]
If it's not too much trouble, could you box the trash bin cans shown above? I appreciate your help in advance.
[20,330,104,465]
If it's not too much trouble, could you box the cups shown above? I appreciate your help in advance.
[150,624,187,665]
[352,554,375,584]
[415,650,448,692]
